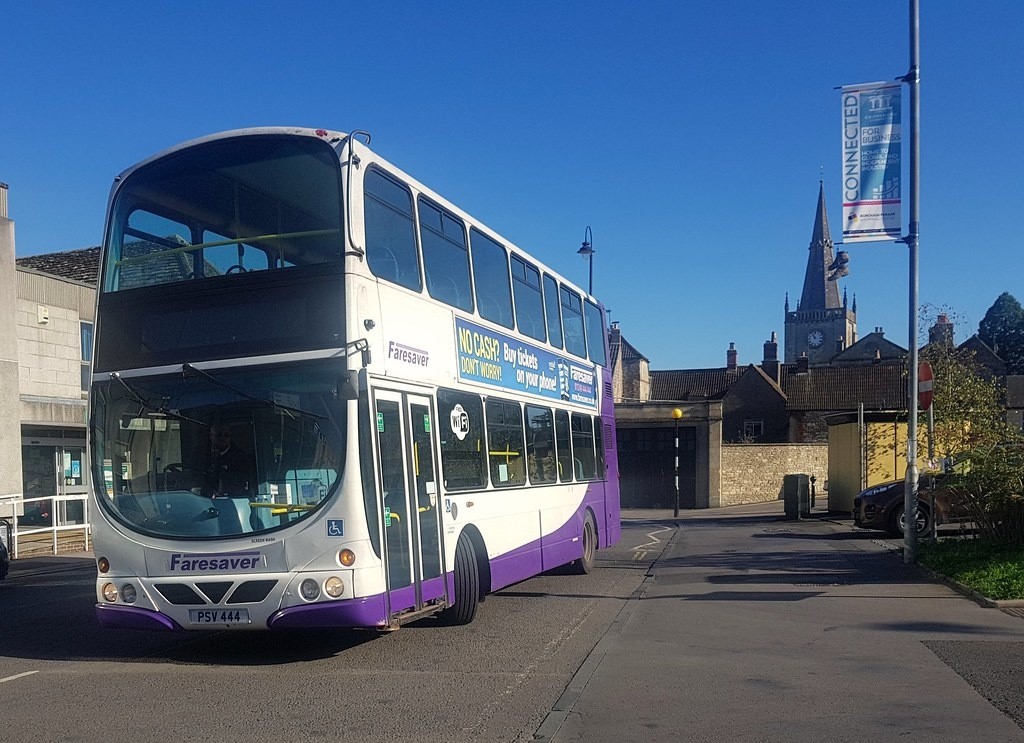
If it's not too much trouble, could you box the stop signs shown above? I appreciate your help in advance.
[917,361,933,410]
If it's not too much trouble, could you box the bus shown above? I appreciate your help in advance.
[83,125,625,646]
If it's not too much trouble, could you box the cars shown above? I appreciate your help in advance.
[849,470,991,538]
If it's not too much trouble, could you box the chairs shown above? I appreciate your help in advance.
[365,246,594,362]
[559,456,584,480]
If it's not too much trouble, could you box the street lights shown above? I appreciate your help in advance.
[670,408,683,517]
[575,225,593,296]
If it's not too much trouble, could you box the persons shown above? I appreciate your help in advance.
[183,418,264,496]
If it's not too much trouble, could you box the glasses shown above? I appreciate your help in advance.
[212,431,232,438]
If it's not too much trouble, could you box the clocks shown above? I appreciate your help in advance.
[805,328,825,349]
